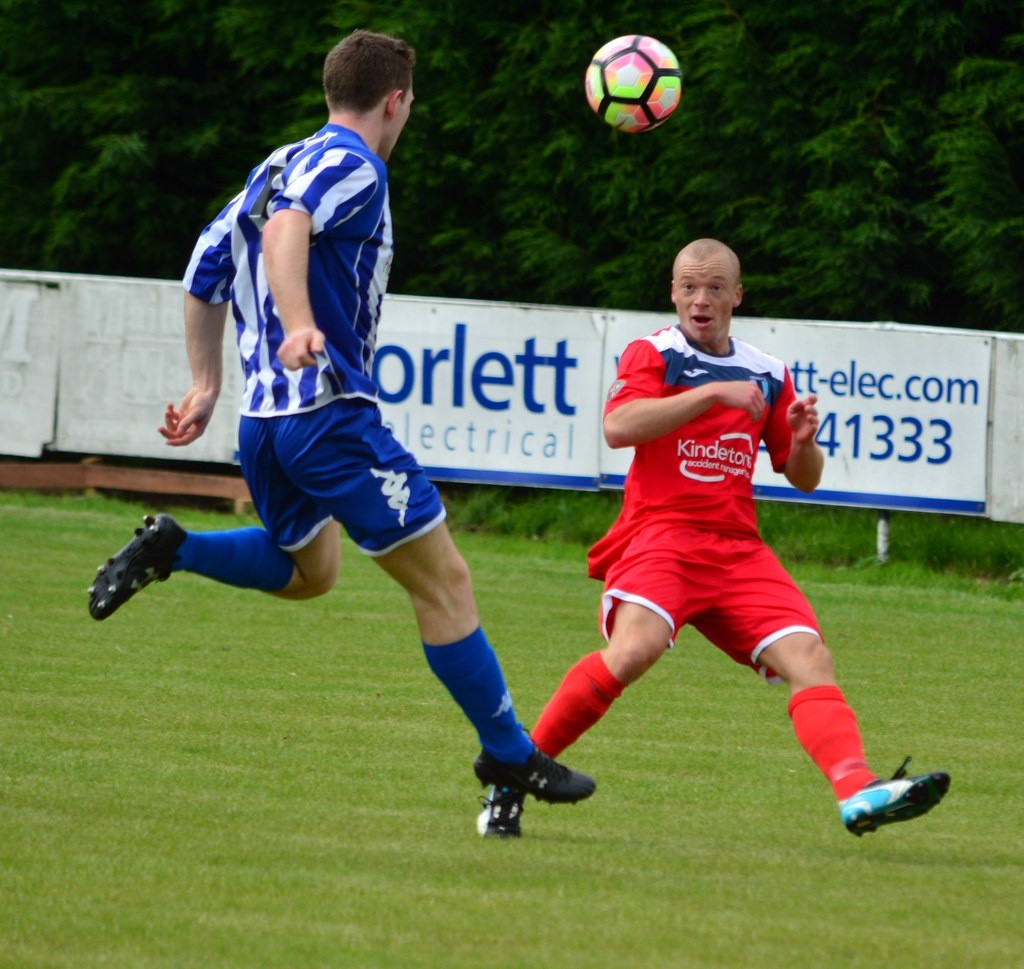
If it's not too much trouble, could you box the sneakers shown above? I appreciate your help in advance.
[474,726,595,806]
[839,755,950,837]
[476,784,526,839]
[87,513,188,621]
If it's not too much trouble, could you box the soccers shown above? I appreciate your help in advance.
[584,33,683,131]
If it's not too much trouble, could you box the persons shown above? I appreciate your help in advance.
[477,236,952,839]
[86,30,597,808]
